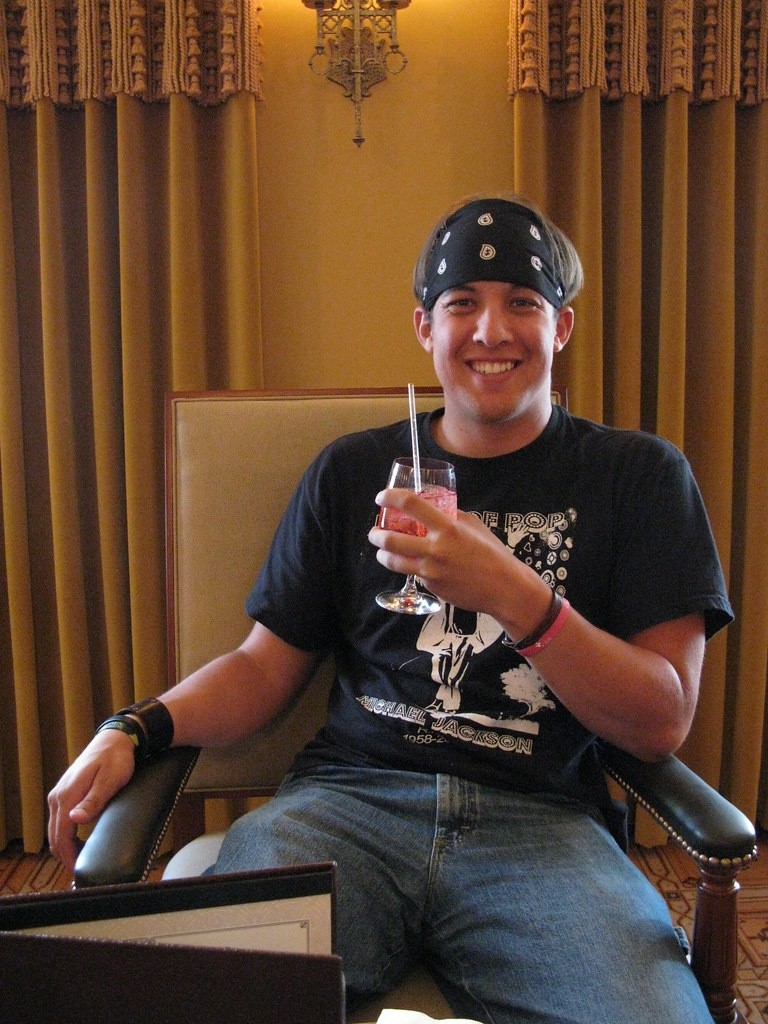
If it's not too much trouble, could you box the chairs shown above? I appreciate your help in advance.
[72,386,758,1024]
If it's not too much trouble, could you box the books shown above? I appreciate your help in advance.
[0,862,347,1023]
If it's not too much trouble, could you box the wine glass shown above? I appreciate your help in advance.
[374,457,457,615]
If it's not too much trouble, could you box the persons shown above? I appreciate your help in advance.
[46,196,735,1024]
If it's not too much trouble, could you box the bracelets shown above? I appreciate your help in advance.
[93,714,147,767]
[114,697,175,754]
[501,587,570,657]
